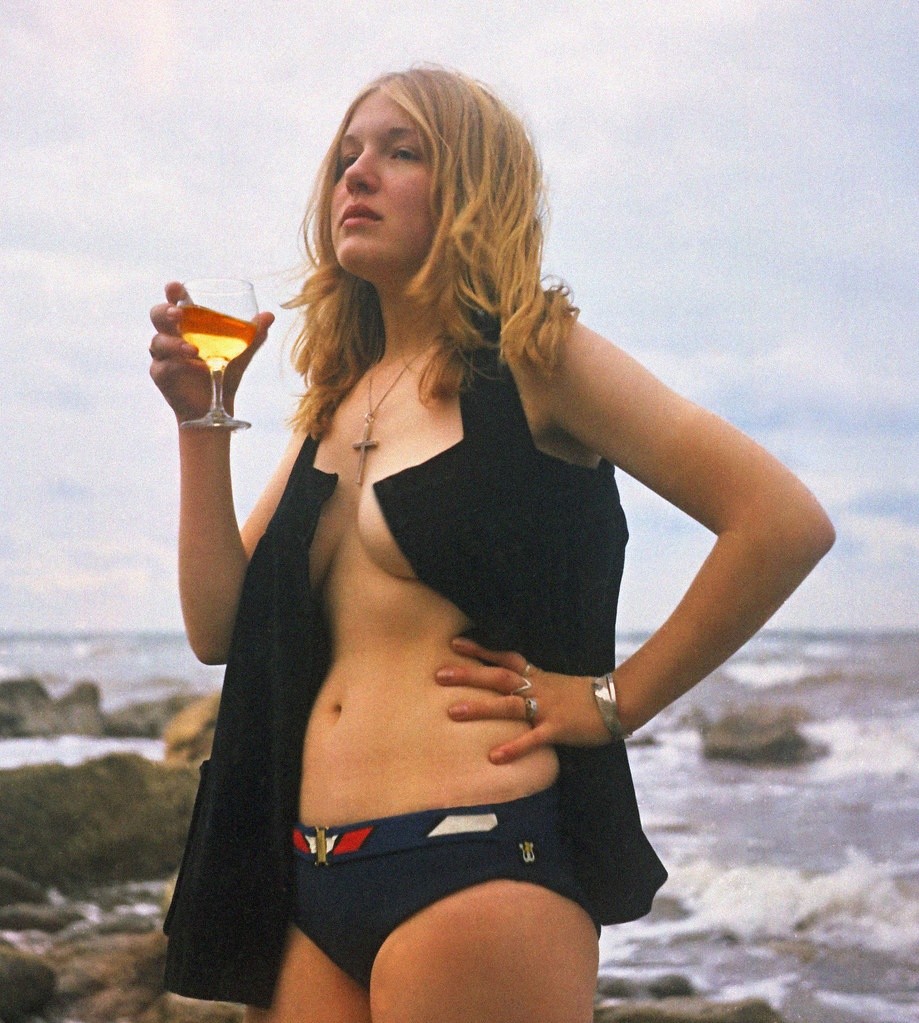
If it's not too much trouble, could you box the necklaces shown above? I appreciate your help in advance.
[353,336,440,485]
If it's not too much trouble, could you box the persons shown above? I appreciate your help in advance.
[150,71,837,1023]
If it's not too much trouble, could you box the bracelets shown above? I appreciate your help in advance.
[592,673,633,741]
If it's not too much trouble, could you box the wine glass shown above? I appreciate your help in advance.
[176,279,259,430]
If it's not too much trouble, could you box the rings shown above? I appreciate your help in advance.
[522,665,530,675]
[514,677,531,694]
[525,697,537,720]
